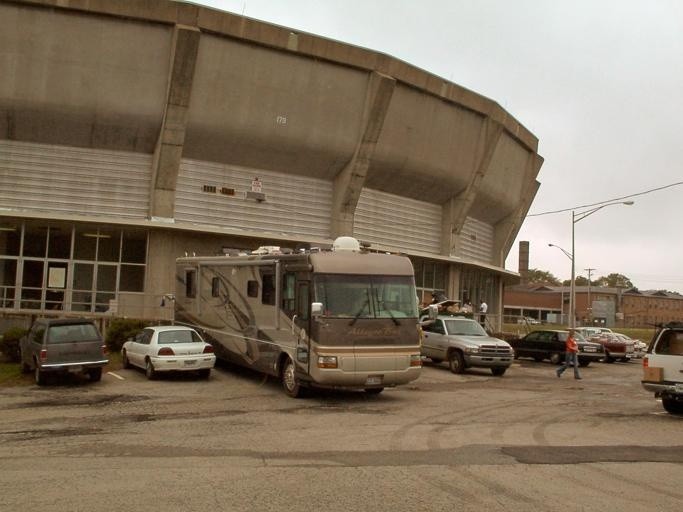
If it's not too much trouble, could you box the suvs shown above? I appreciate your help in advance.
[16,317,109,384]
[640,320,683,416]
[417,315,512,376]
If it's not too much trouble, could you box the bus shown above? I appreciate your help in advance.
[171,235,422,399]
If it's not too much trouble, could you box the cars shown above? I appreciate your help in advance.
[502,312,541,326]
[120,325,217,379]
[510,325,650,368]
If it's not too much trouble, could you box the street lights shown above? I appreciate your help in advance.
[583,267,595,325]
[548,199,635,328]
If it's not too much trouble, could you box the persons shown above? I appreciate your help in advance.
[554,329,583,380]
[463,299,473,314]
[478,299,488,330]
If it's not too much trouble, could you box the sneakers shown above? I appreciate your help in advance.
[575,377,582,379]
[557,370,561,377]
